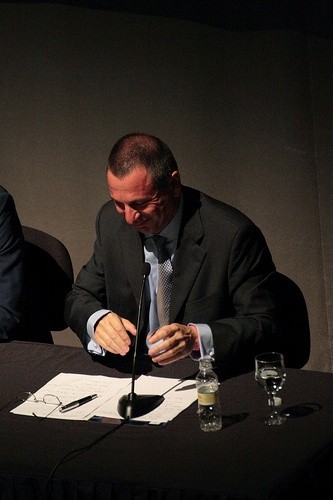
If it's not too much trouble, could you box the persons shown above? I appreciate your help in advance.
[64,132,277,366]
[0,185,54,345]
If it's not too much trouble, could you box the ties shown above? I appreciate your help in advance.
[150,235,172,330]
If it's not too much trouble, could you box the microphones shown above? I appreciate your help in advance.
[117,262,165,418]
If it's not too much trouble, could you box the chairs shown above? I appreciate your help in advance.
[9,225,74,344]
[264,272,311,371]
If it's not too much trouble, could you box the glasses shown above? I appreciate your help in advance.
[0,391,62,421]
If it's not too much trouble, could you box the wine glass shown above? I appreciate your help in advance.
[254,352,288,426]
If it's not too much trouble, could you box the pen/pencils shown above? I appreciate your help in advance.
[59,394,101,413]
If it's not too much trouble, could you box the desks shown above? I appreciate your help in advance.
[0,341,333,500]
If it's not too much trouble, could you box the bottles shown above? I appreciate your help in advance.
[196,358,222,432]
